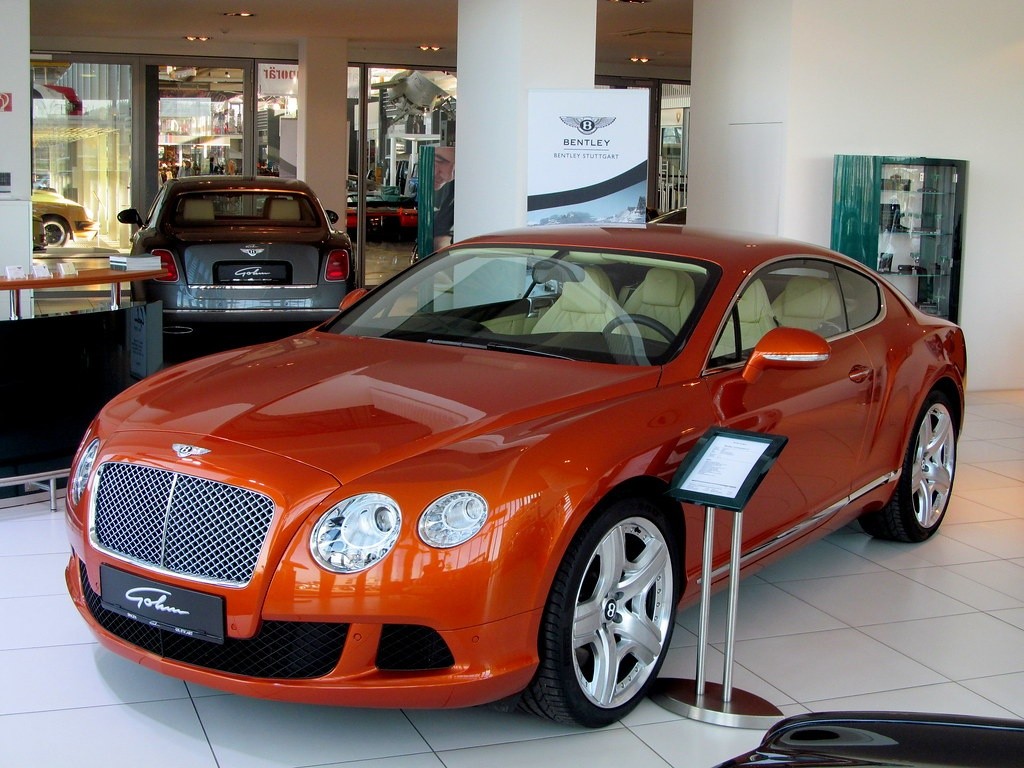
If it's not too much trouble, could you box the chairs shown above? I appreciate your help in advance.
[263,198,301,220]
[531,268,843,358]
[179,196,215,219]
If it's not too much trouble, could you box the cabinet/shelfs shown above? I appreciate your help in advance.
[831,153,969,328]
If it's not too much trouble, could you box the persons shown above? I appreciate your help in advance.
[433,145,455,254]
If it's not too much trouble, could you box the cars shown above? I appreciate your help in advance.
[30,189,94,252]
[116,174,356,364]
[346,174,417,243]
[713,710,1024,768]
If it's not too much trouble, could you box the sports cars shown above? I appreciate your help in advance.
[61,225,968,733]
